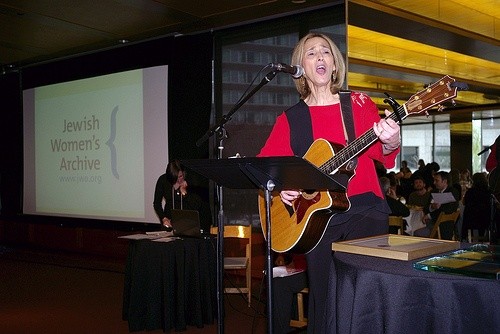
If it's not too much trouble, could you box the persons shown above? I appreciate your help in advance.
[266,253,309,334]
[153,160,206,233]
[257,31,401,334]
[374,136,500,243]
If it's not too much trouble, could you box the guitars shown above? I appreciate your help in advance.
[258,75,459,254]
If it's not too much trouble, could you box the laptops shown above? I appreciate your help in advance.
[170,208,217,238]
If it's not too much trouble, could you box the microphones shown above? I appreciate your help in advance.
[269,62,303,78]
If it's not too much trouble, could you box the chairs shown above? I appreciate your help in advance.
[210,224,252,307]
[389,211,459,238]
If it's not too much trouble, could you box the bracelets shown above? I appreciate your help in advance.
[384,141,401,150]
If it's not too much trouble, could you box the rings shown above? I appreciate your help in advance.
[380,130,383,133]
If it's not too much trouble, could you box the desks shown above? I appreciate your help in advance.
[123,234,227,334]
[403,207,427,236]
[325,243,500,334]
